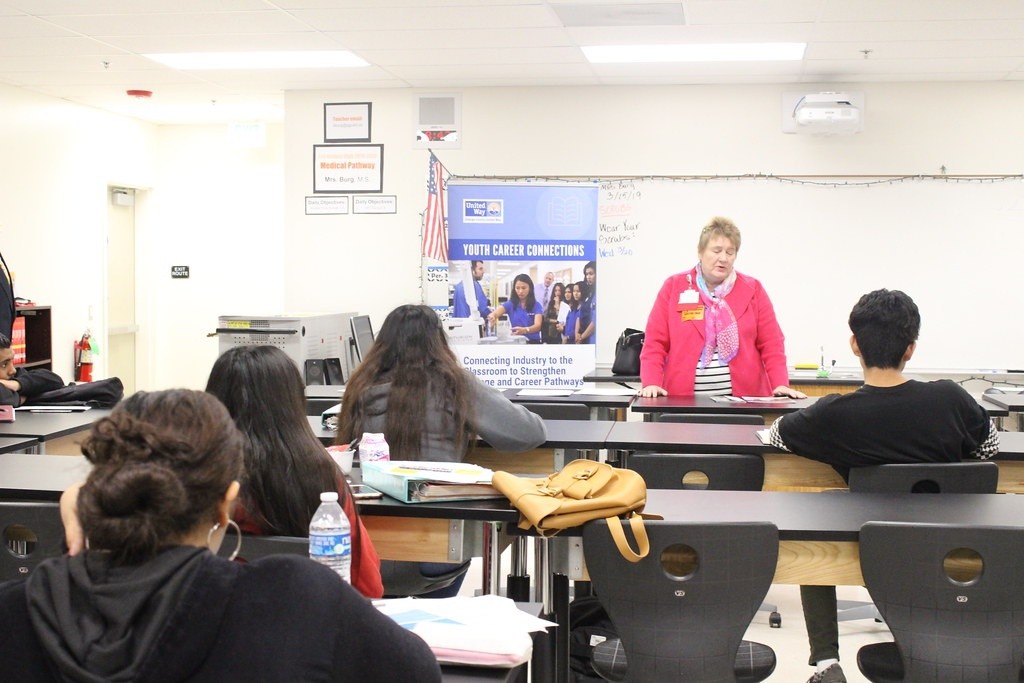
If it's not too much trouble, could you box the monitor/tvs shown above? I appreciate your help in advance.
[345,315,374,380]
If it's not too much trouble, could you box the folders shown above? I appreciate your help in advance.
[361,461,509,502]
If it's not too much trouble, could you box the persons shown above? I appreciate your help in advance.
[0,389,443,683]
[0,333,64,407]
[205,345,384,599]
[638,218,808,398]
[339,306,547,577]
[771,288,1000,683]
[453,260,596,344]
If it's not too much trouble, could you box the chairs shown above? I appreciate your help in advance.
[353,458,472,596]
[628,454,765,491]
[351,314,376,363]
[0,501,65,580]
[520,403,590,420]
[215,530,311,564]
[659,414,765,425]
[493,314,512,336]
[582,518,780,683]
[857,520,1024,683]
[849,462,999,494]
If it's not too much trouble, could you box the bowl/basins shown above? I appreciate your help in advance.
[324,447,356,476]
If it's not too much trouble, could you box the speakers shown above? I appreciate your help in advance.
[306,359,324,386]
[323,358,345,385]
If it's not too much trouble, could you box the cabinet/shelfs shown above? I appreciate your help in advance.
[13,305,53,373]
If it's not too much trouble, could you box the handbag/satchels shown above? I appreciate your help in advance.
[490,459,650,564]
[24,376,124,408]
[610,328,646,377]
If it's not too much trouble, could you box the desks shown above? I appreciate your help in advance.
[365,598,552,683]
[0,367,1024,598]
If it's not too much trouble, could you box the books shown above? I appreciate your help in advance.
[10,318,25,364]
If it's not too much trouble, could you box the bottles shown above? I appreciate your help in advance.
[309,492,351,585]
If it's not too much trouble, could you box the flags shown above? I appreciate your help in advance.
[424,155,448,263]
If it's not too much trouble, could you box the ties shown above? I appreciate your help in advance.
[542,287,548,312]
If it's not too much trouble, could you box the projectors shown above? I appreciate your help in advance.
[796,107,864,126]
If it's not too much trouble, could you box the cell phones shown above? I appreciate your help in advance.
[349,484,383,499]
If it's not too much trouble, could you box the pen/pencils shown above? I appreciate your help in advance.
[346,438,361,452]
[821,345,824,366]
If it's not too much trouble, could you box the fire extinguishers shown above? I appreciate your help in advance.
[75,331,94,383]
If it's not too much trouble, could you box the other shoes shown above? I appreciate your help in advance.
[807,662,847,683]
